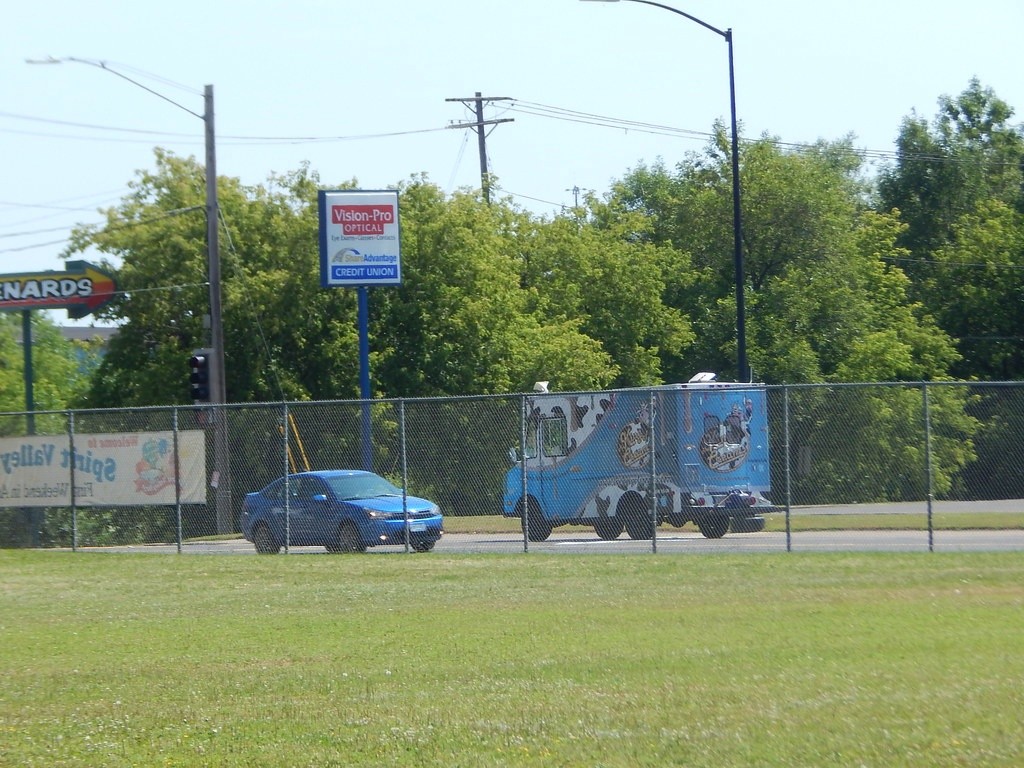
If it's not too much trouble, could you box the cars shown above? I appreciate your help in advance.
[239,469,444,555]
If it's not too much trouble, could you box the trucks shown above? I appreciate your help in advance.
[504,372,785,543]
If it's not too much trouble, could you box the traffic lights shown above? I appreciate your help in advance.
[189,347,212,411]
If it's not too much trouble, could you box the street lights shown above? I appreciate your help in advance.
[25,53,234,534]
[576,1,750,384]
